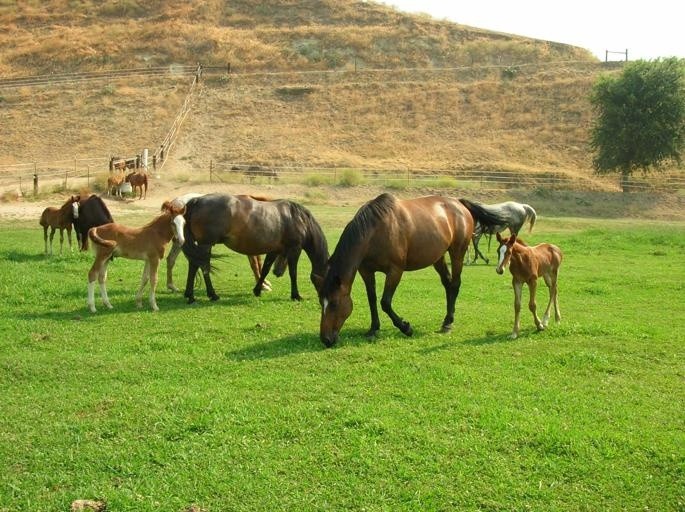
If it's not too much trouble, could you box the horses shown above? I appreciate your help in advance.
[309,191,511,351]
[468,198,538,263]
[105,171,150,197]
[38,192,331,314]
[493,229,563,340]
